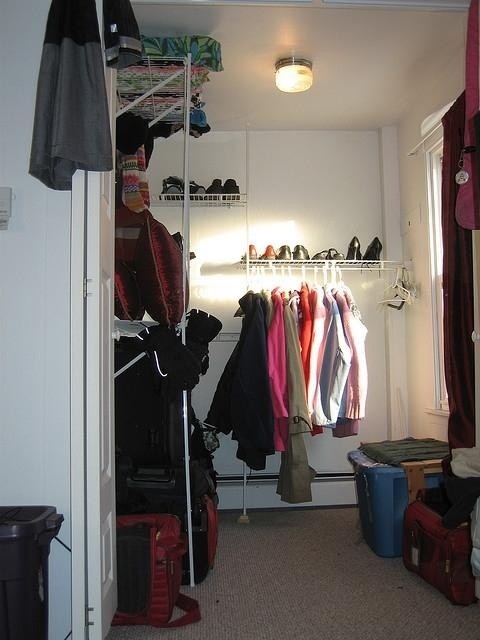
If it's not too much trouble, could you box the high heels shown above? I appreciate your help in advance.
[364,237,383,261]
[346,236,361,260]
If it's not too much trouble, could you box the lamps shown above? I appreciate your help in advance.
[275,57,313,93]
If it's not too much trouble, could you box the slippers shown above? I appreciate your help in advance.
[311,247,344,262]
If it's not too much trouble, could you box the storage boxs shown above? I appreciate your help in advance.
[347,450,442,559]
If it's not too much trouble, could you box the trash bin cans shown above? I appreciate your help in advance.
[0,506,65,640]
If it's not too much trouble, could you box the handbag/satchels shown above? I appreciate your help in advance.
[403,497,474,606]
[122,487,219,585]
[116,513,183,628]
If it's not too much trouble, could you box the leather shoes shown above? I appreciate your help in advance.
[260,245,275,261]
[242,245,258,262]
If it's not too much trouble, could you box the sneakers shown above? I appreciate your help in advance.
[293,245,309,261]
[161,176,184,200]
[206,178,223,197]
[278,245,291,262]
[189,180,204,200]
[224,179,240,200]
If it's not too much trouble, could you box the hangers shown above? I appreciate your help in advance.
[252,265,342,294]
[375,267,417,310]
